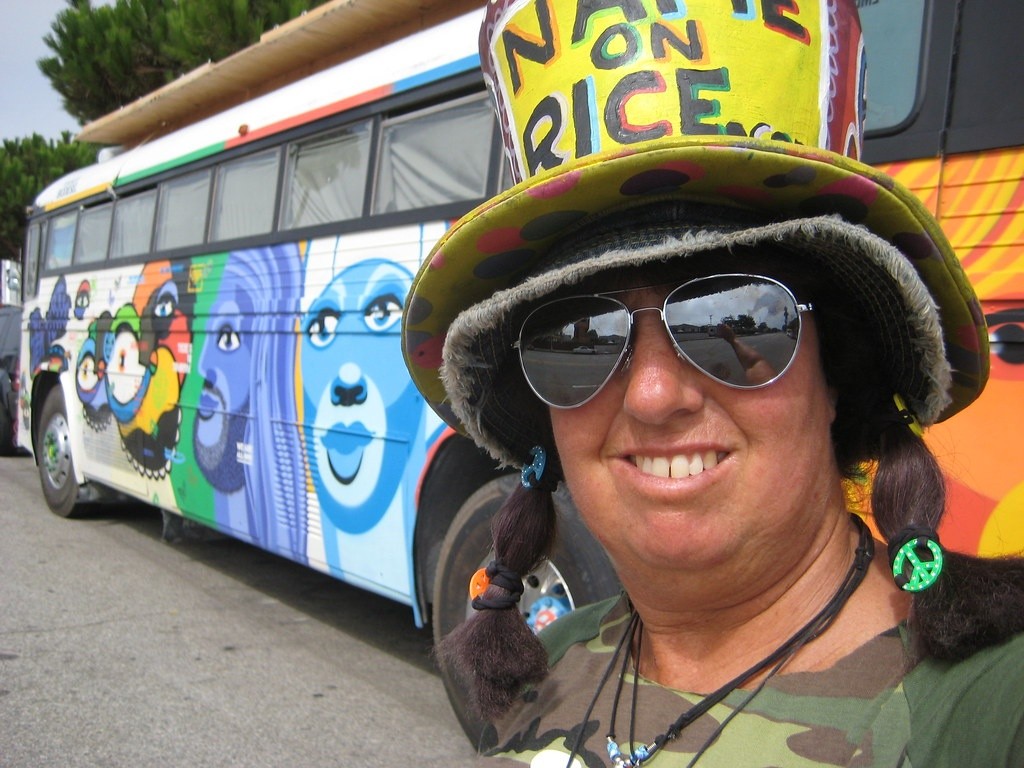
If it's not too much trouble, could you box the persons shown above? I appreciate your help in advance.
[399,0,1024,768]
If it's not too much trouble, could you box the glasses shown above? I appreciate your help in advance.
[510,272,820,409]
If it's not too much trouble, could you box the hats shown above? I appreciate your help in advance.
[401,0,990,441]
[437,205,953,470]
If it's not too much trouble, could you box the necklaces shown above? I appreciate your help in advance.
[564,513,874,768]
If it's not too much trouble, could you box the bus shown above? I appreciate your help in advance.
[14,0,1022,758]
